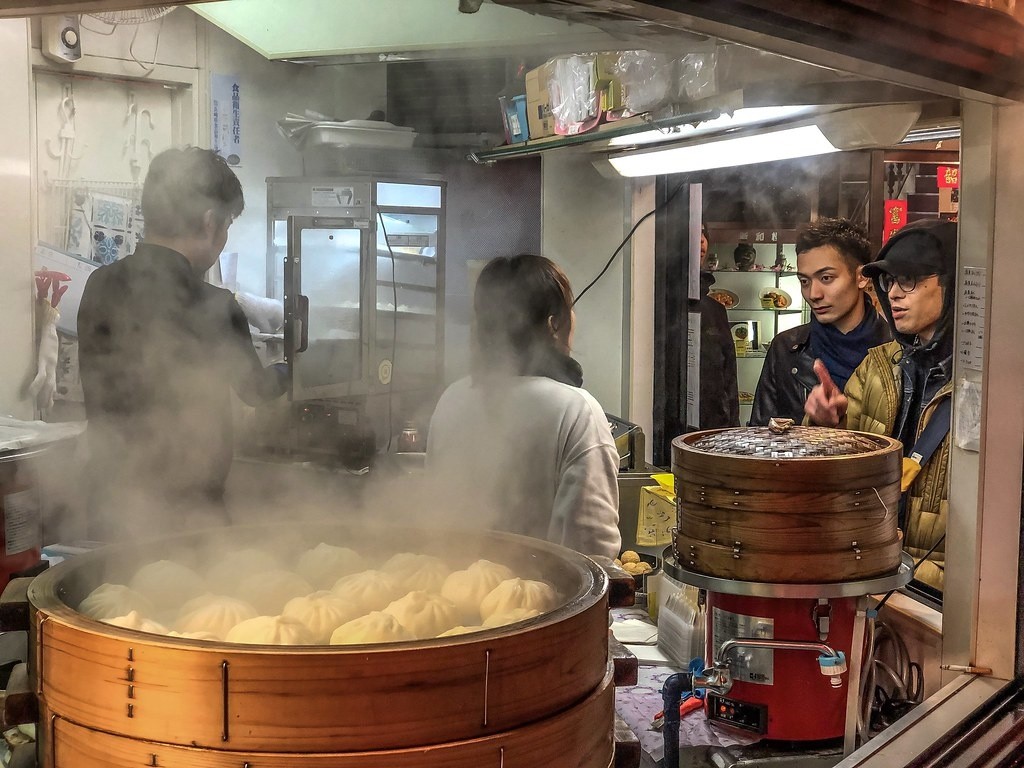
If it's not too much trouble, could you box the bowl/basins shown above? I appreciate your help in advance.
[761,343,772,351]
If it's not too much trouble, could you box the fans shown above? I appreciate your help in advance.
[40,3,178,63]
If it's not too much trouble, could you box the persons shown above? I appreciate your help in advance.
[699,214,960,593]
[421,252,623,563]
[76,145,292,527]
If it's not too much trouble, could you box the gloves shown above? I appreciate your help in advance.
[233,292,284,334]
[264,355,289,367]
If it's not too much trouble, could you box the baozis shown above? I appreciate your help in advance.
[611,551,652,575]
[376,302,409,312]
[75,540,559,647]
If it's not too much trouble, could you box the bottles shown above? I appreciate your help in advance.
[398,420,423,453]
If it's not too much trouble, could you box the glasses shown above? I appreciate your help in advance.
[879,272,941,292]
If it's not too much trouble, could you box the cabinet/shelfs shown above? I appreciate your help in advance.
[703,242,812,424]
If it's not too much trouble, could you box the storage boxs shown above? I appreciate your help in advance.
[502,93,529,144]
[302,125,419,149]
[525,62,557,141]
[607,79,623,109]
[591,54,618,92]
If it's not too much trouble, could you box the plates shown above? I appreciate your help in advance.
[758,287,792,308]
[707,289,740,309]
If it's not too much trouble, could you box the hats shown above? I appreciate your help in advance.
[862,232,947,277]
